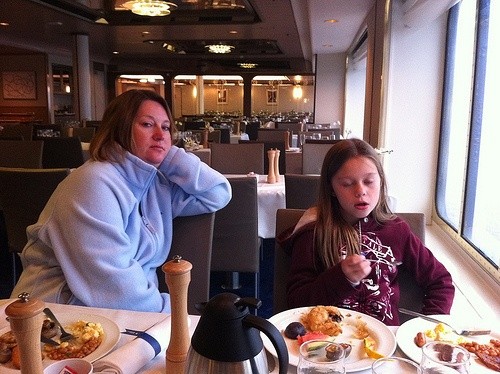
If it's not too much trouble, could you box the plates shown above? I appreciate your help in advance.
[0,311,121,374]
[396,314,500,374]
[259,306,397,373]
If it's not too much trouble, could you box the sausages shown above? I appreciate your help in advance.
[416,332,464,362]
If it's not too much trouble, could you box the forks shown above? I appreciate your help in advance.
[398,308,491,336]
[44,307,79,343]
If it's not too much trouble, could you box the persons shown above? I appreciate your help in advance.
[10,89,232,314]
[274,139,455,325]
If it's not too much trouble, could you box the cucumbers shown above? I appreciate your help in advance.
[306,338,335,352]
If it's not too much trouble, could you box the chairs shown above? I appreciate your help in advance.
[0,123,425,326]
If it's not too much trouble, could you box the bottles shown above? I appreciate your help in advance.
[330,131,336,140]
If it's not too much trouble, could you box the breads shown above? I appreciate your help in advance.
[0,331,21,370]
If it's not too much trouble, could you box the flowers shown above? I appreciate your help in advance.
[344,128,352,139]
[373,145,394,156]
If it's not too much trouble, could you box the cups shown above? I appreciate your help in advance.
[291,134,298,150]
[420,341,471,374]
[321,136,329,140]
[313,132,321,140]
[370,357,422,374]
[173,130,182,148]
[37,129,60,137]
[297,339,346,374]
[305,121,338,129]
[43,358,94,374]
[305,136,312,139]
[299,133,305,146]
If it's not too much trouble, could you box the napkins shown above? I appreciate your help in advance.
[91,314,191,374]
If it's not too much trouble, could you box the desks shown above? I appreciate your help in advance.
[187,145,211,165]
[0,299,420,374]
[221,173,321,238]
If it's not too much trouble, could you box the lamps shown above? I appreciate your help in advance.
[205,44,234,53]
[119,1,177,16]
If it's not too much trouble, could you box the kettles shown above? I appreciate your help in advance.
[182,293,289,374]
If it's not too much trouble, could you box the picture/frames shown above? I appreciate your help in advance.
[266,89,279,105]
[216,88,228,105]
[1,70,37,100]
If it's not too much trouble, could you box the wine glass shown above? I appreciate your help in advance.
[196,133,201,149]
[184,129,192,142]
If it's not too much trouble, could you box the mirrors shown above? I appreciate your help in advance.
[115,74,165,99]
[171,74,244,121]
[250,75,316,124]
